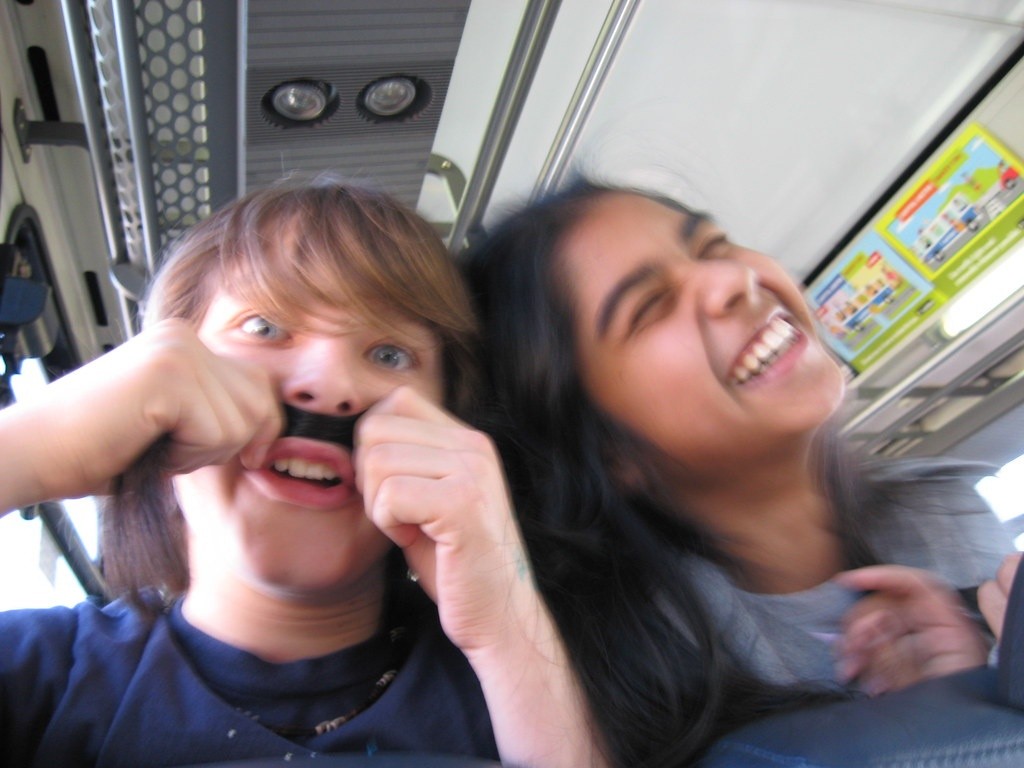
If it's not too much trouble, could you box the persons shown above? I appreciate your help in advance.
[448,184,1024,768]
[0,182,608,768]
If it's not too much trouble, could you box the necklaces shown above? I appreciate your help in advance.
[247,665,398,739]
